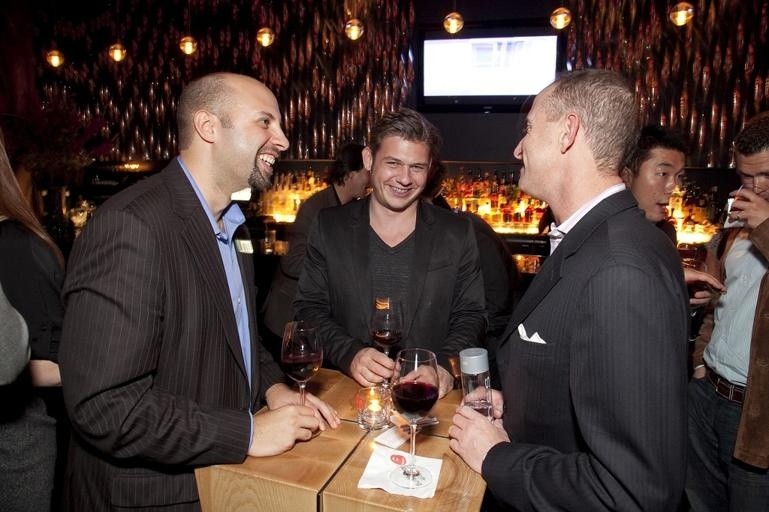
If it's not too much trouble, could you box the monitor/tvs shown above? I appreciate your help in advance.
[416,26,568,115]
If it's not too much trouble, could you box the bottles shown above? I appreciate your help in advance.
[664,174,718,232]
[441,166,545,229]
[460,348,494,424]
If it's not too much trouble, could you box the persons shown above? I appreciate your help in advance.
[619,124,727,308]
[57,72,341,512]
[685,112,769,512]
[1,142,67,512]
[293,108,490,400]
[262,143,371,362]
[431,192,517,348]
[447,68,690,512]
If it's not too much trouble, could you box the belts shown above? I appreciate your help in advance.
[706,366,747,406]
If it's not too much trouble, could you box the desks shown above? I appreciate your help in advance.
[192,364,499,512]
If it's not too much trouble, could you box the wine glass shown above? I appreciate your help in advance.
[368,297,440,489]
[281,321,323,438]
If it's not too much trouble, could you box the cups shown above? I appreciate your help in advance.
[355,386,390,431]
